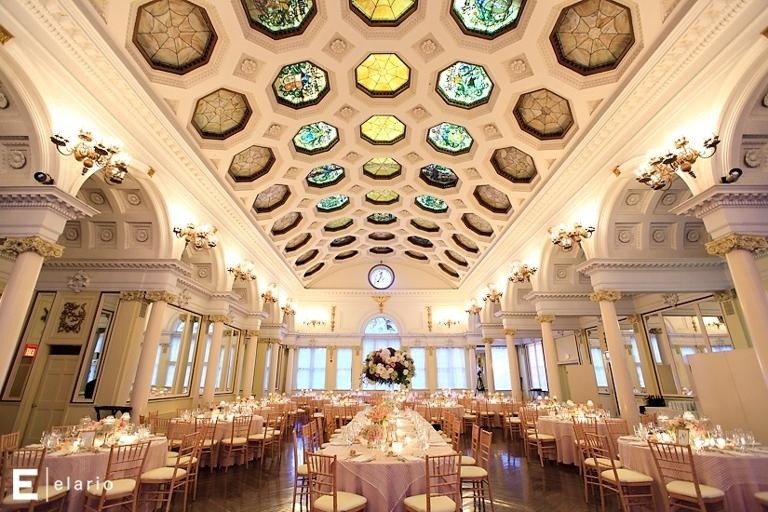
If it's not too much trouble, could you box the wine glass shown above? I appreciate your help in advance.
[334,404,433,461]
[21,413,150,453]
[177,404,252,423]
[528,396,610,423]
[634,412,768,457]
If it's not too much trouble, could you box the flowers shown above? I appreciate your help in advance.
[358,346,417,389]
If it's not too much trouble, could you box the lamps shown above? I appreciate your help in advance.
[465,302,482,316]
[633,126,720,193]
[171,220,217,250]
[261,288,279,304]
[482,288,504,305]
[507,264,538,285]
[48,122,128,189]
[282,302,296,317]
[227,262,256,282]
[546,219,595,253]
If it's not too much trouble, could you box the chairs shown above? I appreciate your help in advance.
[0,388,766,511]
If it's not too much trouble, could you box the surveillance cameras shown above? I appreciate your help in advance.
[33,172,54,185]
[722,168,742,183]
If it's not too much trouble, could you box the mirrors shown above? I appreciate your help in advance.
[583,326,610,396]
[70,290,126,405]
[132,299,203,402]
[198,317,243,395]
[617,316,649,396]
[1,289,61,402]
[639,289,735,401]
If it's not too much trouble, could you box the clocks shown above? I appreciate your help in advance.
[367,259,396,292]
